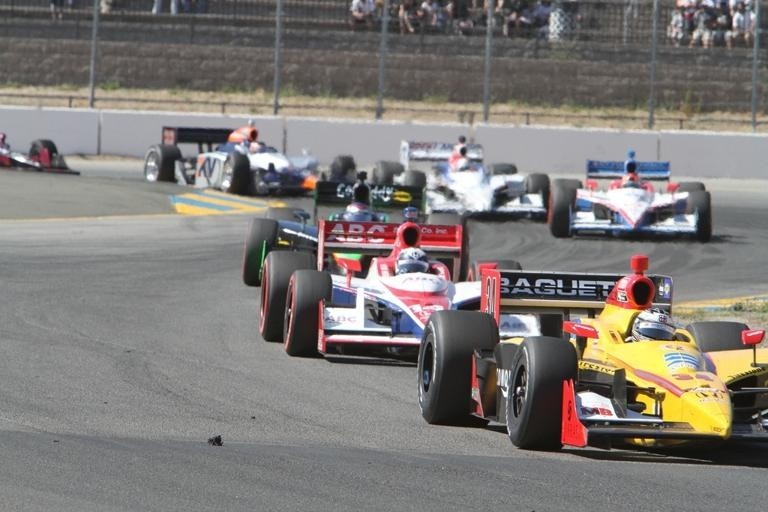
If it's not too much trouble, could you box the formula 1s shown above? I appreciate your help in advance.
[143,119,305,198]
[0,131,80,175]
[258,207,522,365]
[416,254,768,452]
[143,118,768,450]
[243,171,427,287]
[398,135,551,224]
[312,155,429,215]
[548,151,714,244]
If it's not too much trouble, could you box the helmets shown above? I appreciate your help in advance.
[623,173,639,188]
[456,159,469,172]
[343,203,369,221]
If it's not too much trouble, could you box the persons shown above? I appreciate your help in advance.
[343,202,372,222]
[623,173,639,183]
[49,0,65,21]
[346,0,583,41]
[666,0,759,50]
[457,157,471,172]
[150,0,189,15]
